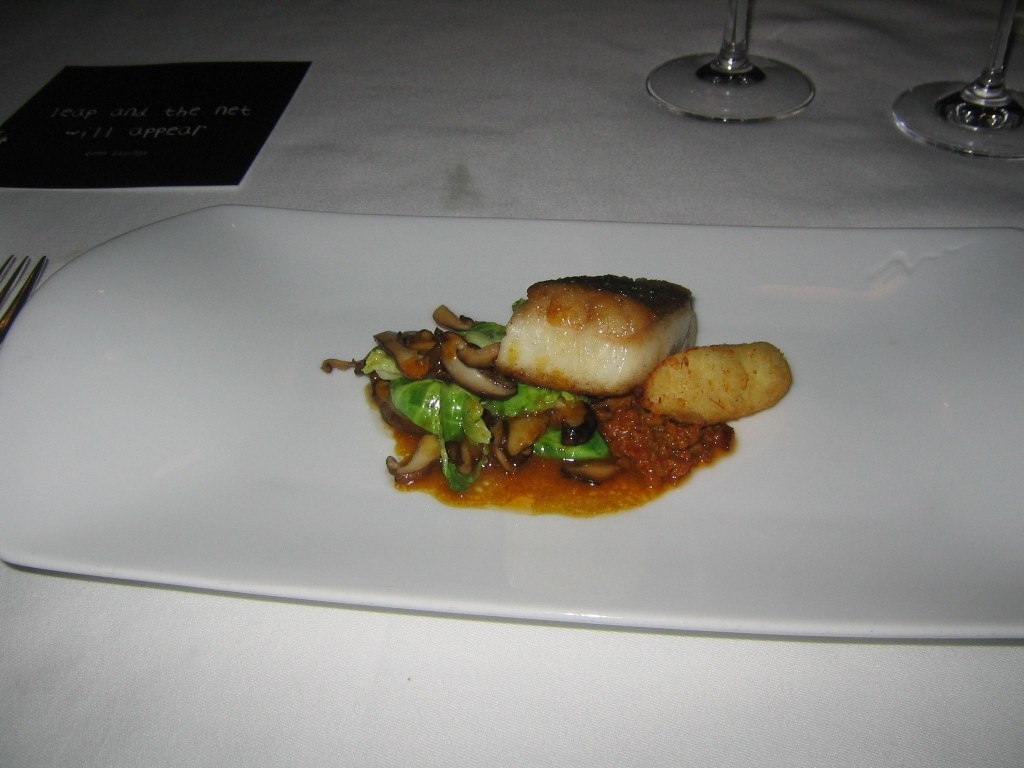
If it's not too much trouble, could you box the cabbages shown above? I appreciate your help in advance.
[357,298,612,491]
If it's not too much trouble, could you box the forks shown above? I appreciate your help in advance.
[0,251,50,347]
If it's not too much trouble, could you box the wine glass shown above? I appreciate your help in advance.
[891,0,1024,160]
[643,0,817,124]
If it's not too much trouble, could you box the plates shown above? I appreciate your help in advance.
[1,201,1024,643]
